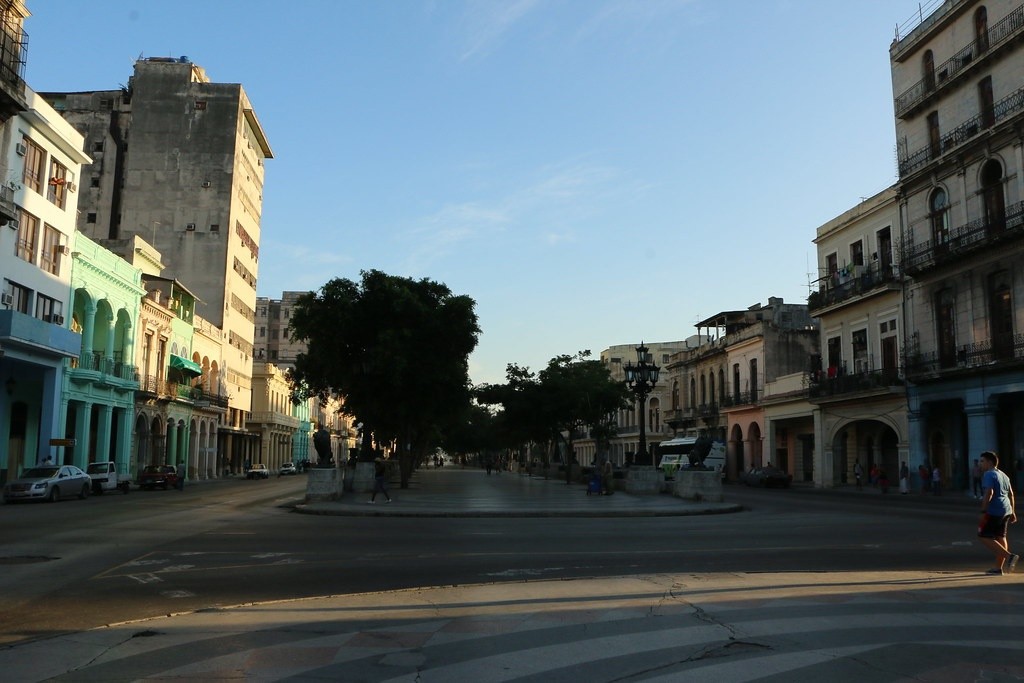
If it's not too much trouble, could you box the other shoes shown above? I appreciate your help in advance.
[973,496,977,500]
[1007,553,1019,574]
[986,568,1003,575]
[979,497,983,500]
[367,499,375,504]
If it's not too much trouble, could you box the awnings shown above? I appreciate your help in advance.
[170,353,203,379]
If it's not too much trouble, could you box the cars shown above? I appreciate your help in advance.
[3,464,92,503]
[280,463,295,473]
[139,464,176,491]
[743,467,792,488]
[247,463,269,478]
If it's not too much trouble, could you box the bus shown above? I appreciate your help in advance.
[657,437,727,480]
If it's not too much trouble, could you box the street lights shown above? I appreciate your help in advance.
[623,340,661,464]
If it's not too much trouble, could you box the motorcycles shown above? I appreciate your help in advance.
[86,461,132,495]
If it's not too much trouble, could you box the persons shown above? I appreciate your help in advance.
[977,452,1020,576]
[929,466,943,496]
[433,453,444,467]
[175,459,186,492]
[852,457,890,494]
[973,458,984,500]
[532,458,540,476]
[297,457,311,471]
[485,454,503,474]
[899,461,909,495]
[367,457,391,503]
[223,458,233,475]
[39,455,53,467]
[749,464,754,469]
[599,458,615,496]
[767,462,774,469]
